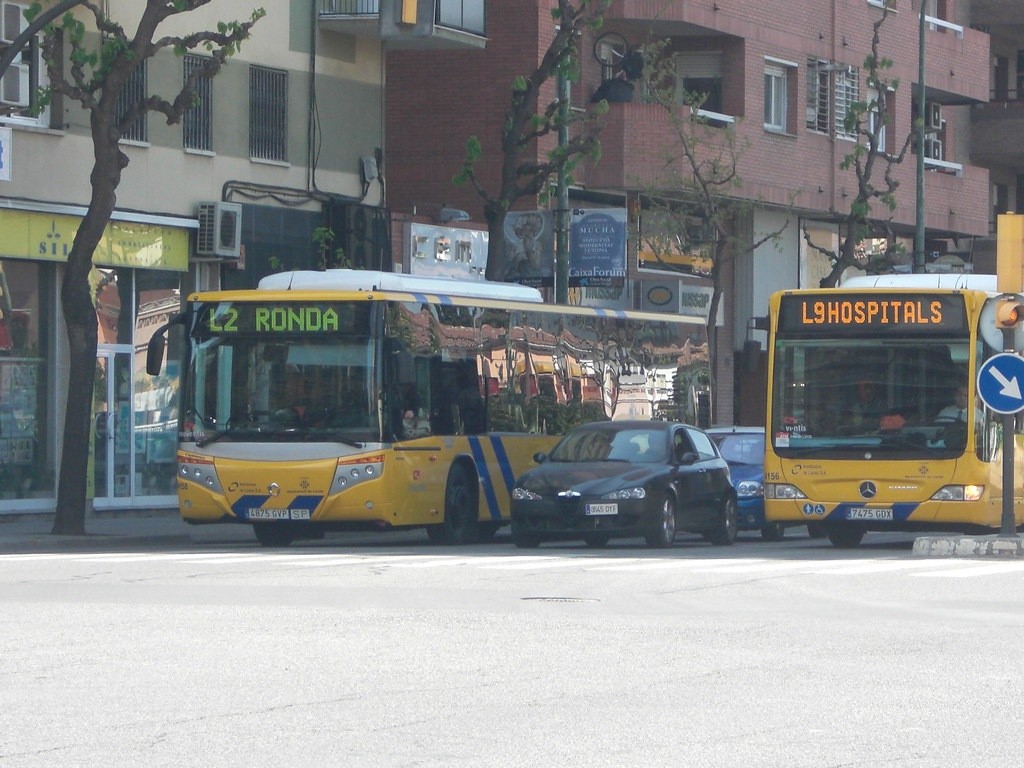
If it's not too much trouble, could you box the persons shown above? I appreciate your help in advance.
[643,432,668,463]
[926,382,985,448]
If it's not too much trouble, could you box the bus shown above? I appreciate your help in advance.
[763,270,1024,545]
[143,266,716,547]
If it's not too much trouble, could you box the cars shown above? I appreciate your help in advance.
[703,426,767,536]
[510,419,738,550]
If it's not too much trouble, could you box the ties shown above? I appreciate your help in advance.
[956,410,963,422]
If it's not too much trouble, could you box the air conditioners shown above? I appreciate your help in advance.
[196,201,242,257]
[345,205,373,269]
[0,62,30,111]
[915,101,942,133]
[925,139,942,161]
